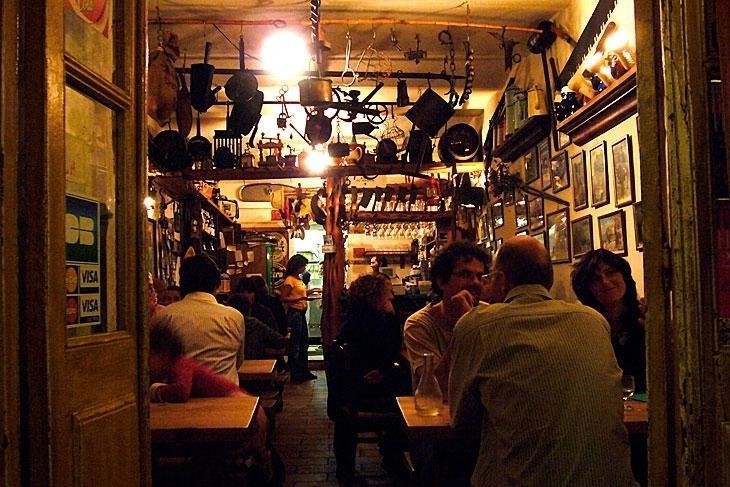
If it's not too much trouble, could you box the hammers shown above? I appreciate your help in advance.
[527,20,560,152]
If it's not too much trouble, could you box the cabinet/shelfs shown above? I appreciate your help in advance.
[185,197,234,261]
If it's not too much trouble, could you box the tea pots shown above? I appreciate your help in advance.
[504,84,548,134]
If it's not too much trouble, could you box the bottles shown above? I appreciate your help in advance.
[413,353,443,418]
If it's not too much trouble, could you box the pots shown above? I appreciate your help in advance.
[298,78,334,105]
[225,34,258,104]
[187,113,212,161]
[438,124,481,164]
[149,120,189,172]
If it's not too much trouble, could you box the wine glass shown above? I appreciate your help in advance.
[621,376,636,411]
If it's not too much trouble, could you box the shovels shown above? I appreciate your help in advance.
[190,42,215,112]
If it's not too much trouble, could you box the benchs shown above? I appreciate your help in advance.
[403,450,415,480]
[254,375,288,411]
[331,409,402,479]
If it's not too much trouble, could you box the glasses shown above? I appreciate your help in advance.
[481,271,497,281]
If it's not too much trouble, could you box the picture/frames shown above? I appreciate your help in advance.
[460,134,644,265]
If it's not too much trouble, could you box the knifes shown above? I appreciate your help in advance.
[350,186,418,216]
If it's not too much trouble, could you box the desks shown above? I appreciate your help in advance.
[149,397,261,442]
[394,395,648,441]
[237,359,277,381]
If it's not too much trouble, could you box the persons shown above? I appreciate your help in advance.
[333,236,647,485]
[145,254,323,485]
